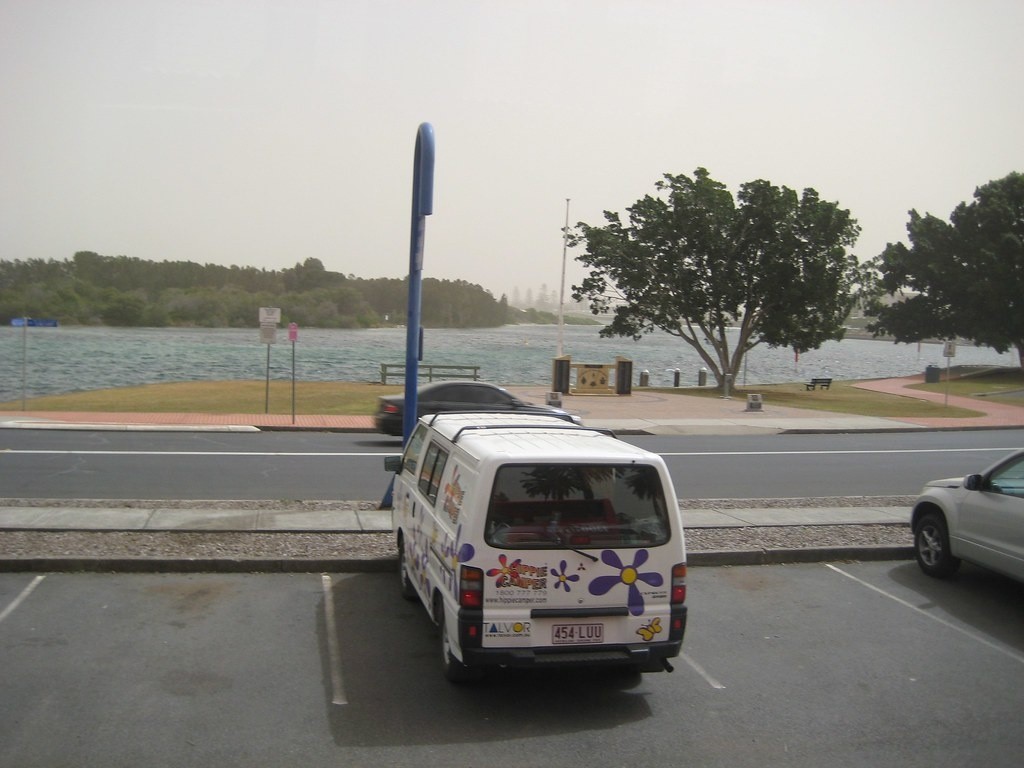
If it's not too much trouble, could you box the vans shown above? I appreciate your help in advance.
[382,408,690,686]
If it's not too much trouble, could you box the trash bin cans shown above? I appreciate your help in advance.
[926,364,940,382]
[698,367,707,386]
[640,369,649,386]
[551,358,571,395]
[616,360,633,395]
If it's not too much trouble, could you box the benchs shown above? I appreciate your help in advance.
[804,378,832,391]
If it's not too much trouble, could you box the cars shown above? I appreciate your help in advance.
[910,447,1023,584]
[373,379,583,436]
